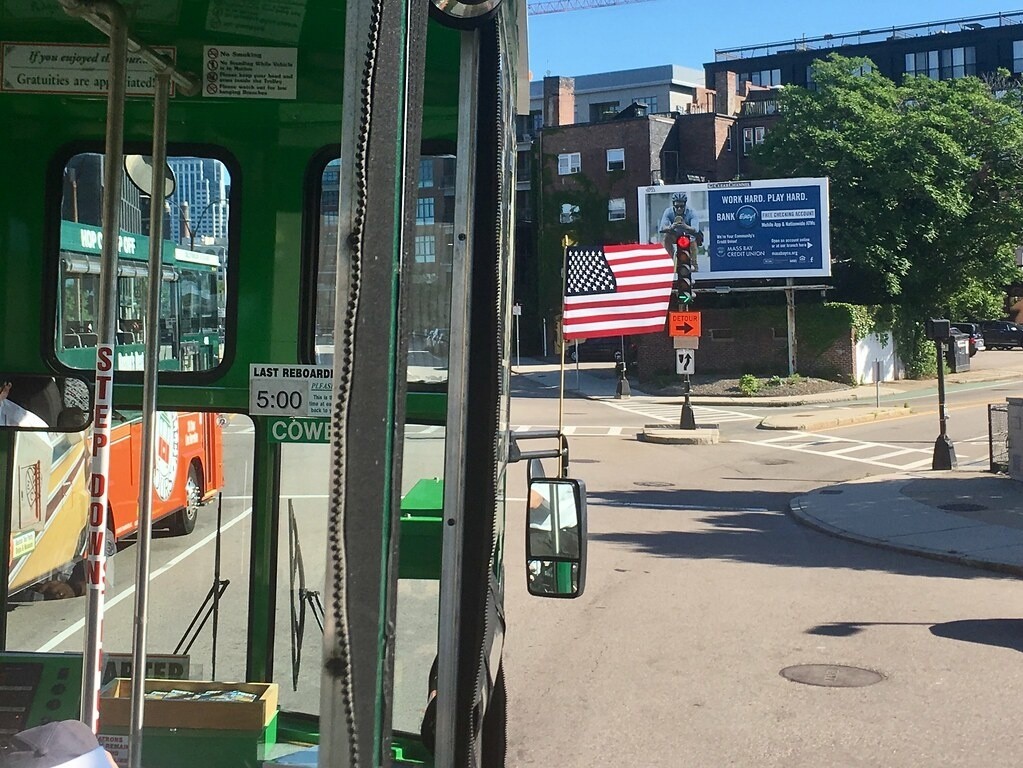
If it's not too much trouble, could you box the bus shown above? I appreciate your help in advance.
[1,219,225,593]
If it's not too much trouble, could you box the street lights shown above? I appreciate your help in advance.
[140,195,229,252]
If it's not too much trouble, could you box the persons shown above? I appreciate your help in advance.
[659,193,699,272]
[0,375,49,428]
[529,483,579,558]
[8,560,86,602]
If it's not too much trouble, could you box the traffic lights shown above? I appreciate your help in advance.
[678,235,691,304]
[925,320,948,340]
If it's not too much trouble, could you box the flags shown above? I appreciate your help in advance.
[563,243,674,340]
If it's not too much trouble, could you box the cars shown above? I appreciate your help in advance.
[424,330,451,356]
[950,323,985,358]
[976,321,1023,350]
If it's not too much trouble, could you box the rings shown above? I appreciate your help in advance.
[58,590,65,599]
